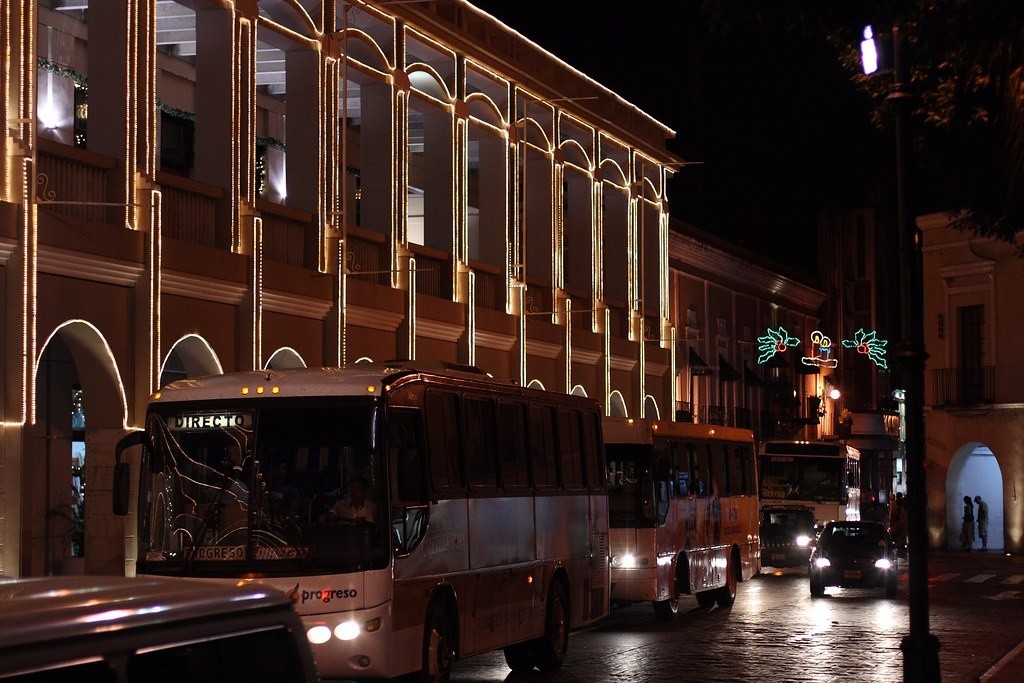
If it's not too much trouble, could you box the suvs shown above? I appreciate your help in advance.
[757,504,816,574]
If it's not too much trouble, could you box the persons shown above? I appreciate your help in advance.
[316,469,387,527]
[887,492,915,549]
[961,496,988,553]
[847,469,854,488]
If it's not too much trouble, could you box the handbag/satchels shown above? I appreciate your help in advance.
[965,513,974,522]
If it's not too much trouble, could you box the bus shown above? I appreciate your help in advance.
[111,362,611,682]
[601,417,762,624]
[756,439,863,528]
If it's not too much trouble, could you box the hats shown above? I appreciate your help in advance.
[974,497,981,502]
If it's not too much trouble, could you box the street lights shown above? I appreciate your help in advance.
[856,16,942,683]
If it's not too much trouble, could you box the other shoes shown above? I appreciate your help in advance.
[978,547,987,552]
[962,545,972,552]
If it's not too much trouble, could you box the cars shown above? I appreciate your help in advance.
[808,520,899,600]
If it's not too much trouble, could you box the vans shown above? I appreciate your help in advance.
[1,573,317,683]
